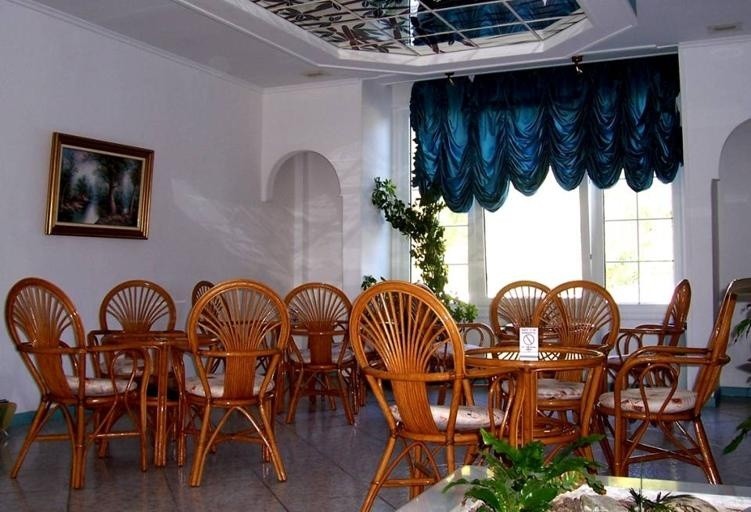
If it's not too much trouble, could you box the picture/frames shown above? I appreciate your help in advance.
[44,131,154,239]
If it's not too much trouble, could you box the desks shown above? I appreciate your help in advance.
[396,465,751,512]
